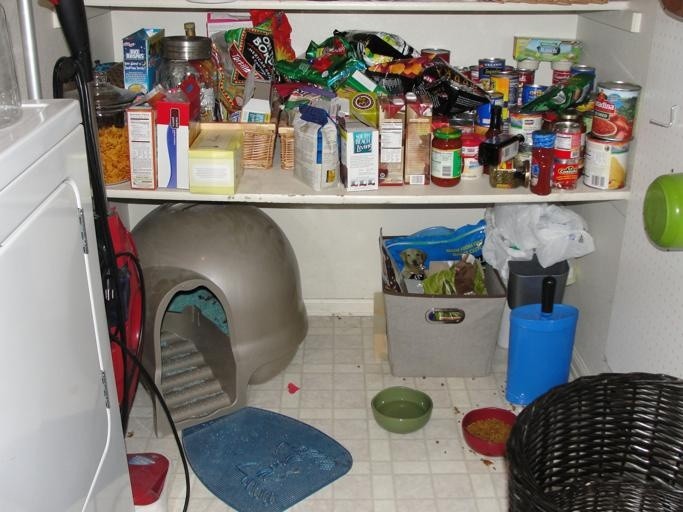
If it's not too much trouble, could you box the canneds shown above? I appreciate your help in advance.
[420,49,642,196]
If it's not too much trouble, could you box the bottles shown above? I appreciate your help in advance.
[183,22,195,39]
[477,132,525,165]
[86,72,138,185]
[531,130,552,195]
[0,2,22,128]
[483,105,503,174]
[156,37,216,122]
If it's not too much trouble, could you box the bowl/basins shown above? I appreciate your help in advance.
[640,174,683,251]
[127,452,169,505]
[462,406,520,457]
[371,386,432,433]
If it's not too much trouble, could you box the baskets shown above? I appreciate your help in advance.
[507,372,682,512]
[199,100,295,169]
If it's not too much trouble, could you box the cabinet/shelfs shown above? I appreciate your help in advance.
[1,98,137,510]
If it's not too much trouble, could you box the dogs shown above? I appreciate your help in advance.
[400,248,428,280]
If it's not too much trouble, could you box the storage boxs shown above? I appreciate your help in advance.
[382,251,507,381]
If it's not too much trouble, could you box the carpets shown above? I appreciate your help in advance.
[177,407,352,512]
